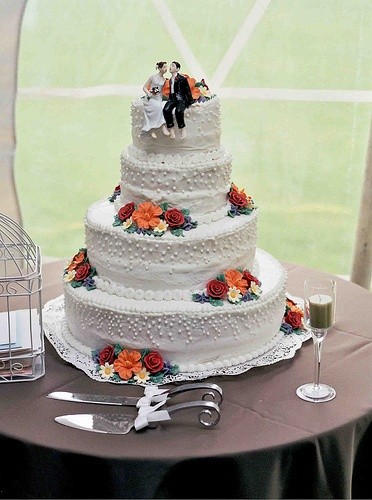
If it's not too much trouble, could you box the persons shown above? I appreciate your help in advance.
[142,61,170,138]
[163,62,194,139]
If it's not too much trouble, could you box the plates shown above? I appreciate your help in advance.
[0,308,42,376]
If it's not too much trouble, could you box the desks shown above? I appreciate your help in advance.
[0,260,372,500]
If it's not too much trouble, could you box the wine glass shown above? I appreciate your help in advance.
[295,277,337,403]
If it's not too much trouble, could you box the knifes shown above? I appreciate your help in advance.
[46,383,226,406]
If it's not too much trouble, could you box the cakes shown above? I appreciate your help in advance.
[61,95,287,374]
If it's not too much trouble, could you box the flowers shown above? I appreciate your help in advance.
[280,296,306,336]
[110,185,122,201]
[91,344,178,385]
[146,74,216,106]
[227,181,257,219]
[113,197,197,240]
[62,247,97,292]
[192,265,262,307]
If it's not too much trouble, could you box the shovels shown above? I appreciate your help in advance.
[54,400,221,435]
